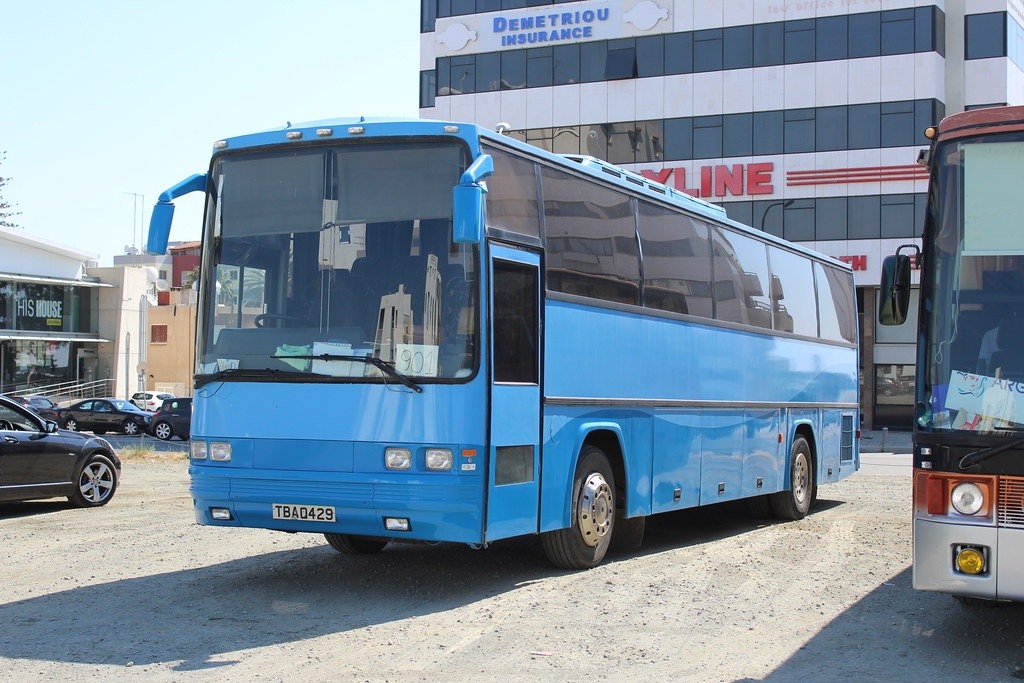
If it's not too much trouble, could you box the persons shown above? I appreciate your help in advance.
[99,402,110,410]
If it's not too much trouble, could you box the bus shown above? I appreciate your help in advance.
[878,105,1024,602]
[148,116,861,571]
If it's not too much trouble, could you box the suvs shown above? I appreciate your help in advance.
[131,391,176,411]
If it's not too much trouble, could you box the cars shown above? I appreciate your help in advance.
[9,396,59,423]
[0,395,121,506]
[150,398,192,441]
[57,399,149,435]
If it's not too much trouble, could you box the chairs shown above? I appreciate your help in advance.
[954,308,998,372]
[304,255,463,328]
